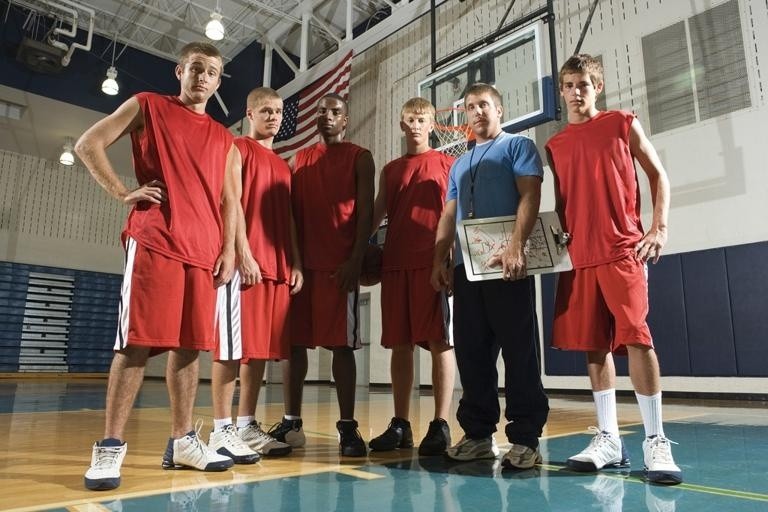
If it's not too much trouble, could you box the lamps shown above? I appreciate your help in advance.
[99,32,121,97]
[59,136,78,166]
[204,0,227,42]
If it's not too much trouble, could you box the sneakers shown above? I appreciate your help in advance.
[336,419,367,457]
[500,443,543,470]
[418,421,451,456]
[160,431,234,472]
[641,435,683,484]
[84,438,127,490]
[207,428,260,465]
[567,433,631,472]
[369,418,414,452]
[270,418,307,449]
[238,421,291,458]
[445,436,496,461]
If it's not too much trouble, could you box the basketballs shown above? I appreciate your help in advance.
[360,245,382,286]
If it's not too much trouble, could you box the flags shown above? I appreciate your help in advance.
[268,49,353,174]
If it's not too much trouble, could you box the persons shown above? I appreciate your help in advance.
[546,53,682,484]
[367,100,459,454]
[428,82,551,471]
[208,89,304,466]
[269,93,373,458]
[73,43,234,491]
